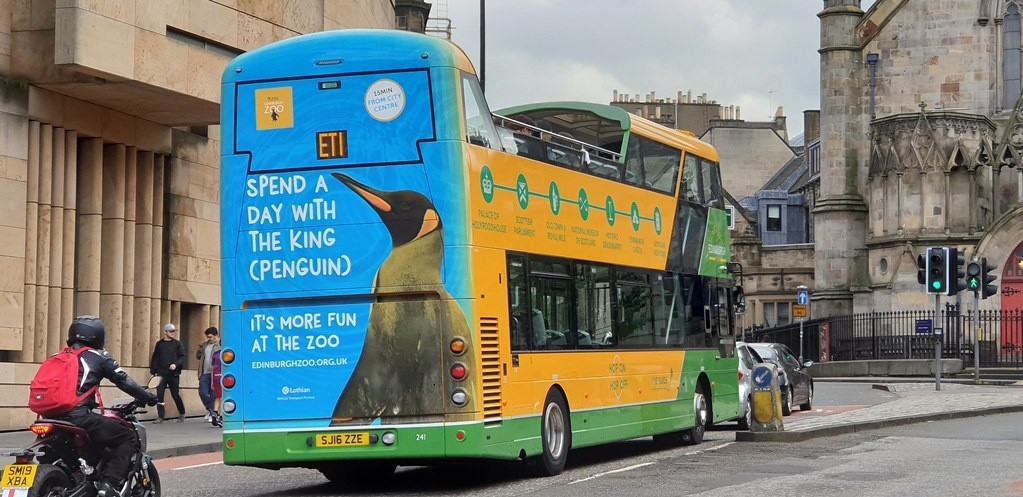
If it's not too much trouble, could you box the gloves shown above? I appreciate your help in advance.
[148,394,158,407]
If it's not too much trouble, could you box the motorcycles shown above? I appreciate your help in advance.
[0,375,165,497]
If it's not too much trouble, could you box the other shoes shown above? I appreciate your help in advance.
[177,414,185,422]
[98,479,121,497]
[153,417,165,424]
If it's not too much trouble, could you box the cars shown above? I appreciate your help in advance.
[747,341,814,416]
[733,341,784,430]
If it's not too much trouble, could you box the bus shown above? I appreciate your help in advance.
[219,28,744,488]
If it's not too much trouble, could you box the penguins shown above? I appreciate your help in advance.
[328,172,476,426]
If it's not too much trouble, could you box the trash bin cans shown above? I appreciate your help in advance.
[979,340,997,367]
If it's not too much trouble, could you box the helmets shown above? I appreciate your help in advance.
[66,314,105,349]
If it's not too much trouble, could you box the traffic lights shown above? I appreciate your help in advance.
[917,254,927,285]
[947,247,967,296]
[967,260,981,291]
[926,248,950,295]
[978,257,999,299]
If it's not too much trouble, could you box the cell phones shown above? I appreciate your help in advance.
[199,345,202,347]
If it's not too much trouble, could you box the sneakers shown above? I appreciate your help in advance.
[212,412,218,426]
[204,412,213,423]
[217,418,224,427]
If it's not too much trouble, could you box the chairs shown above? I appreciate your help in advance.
[512,304,607,349]
[470,133,653,188]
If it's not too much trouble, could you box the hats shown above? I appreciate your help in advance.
[164,324,176,331]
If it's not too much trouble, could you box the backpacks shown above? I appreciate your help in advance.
[28,347,105,420]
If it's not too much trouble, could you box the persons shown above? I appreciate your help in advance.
[508,115,581,168]
[150,324,187,424]
[210,339,223,427]
[196,326,221,422]
[43,315,160,497]
